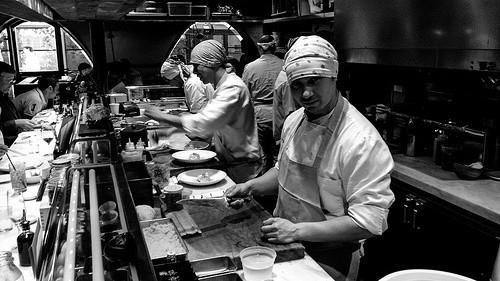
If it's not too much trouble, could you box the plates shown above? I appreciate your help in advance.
[179,168,227,185]
[169,140,209,150]
[0,156,40,171]
[172,150,217,163]
[487,171,500,180]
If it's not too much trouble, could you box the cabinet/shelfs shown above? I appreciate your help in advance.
[371,179,500,281]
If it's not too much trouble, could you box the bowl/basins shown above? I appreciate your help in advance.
[26,169,39,183]
[454,163,485,180]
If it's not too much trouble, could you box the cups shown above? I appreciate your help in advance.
[99,201,118,224]
[164,185,184,210]
[9,162,27,194]
[239,246,277,281]
[0,206,12,233]
[7,188,25,224]
[109,104,119,114]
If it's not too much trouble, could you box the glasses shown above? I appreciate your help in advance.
[0,74,16,85]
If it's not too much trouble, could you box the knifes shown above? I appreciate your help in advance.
[176,194,254,207]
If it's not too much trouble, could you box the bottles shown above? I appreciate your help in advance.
[46,158,73,204]
[125,137,145,151]
[0,251,24,281]
[16,221,36,267]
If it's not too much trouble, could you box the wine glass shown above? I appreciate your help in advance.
[41,125,55,156]
[52,99,62,120]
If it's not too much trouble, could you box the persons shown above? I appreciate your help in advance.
[119,59,144,98]
[224,35,395,281]
[242,36,299,160]
[142,40,266,186]
[161,54,215,114]
[67,63,95,104]
[12,75,60,120]
[0,61,36,149]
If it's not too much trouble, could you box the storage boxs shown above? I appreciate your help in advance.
[164,1,208,17]
